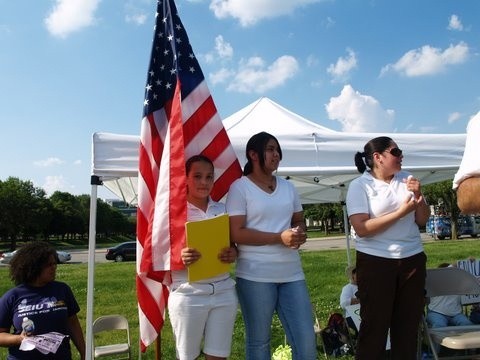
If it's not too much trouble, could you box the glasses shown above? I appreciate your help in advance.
[383,148,403,157]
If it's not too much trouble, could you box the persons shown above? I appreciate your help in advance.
[0,241,87,360]
[163,155,237,360]
[453,107,480,214]
[224,132,322,360]
[426,255,478,360]
[346,138,431,360]
[339,264,393,355]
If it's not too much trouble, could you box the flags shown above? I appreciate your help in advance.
[135,0,244,354]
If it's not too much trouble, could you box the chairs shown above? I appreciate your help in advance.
[284,303,330,360]
[418,268,480,360]
[92,314,131,360]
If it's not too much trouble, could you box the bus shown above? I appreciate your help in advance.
[427,204,480,240]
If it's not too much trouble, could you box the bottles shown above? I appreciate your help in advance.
[22,317,35,336]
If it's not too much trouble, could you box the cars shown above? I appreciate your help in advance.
[105,241,136,263]
[0,247,71,264]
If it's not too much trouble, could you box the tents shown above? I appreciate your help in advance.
[82,99,467,360]
